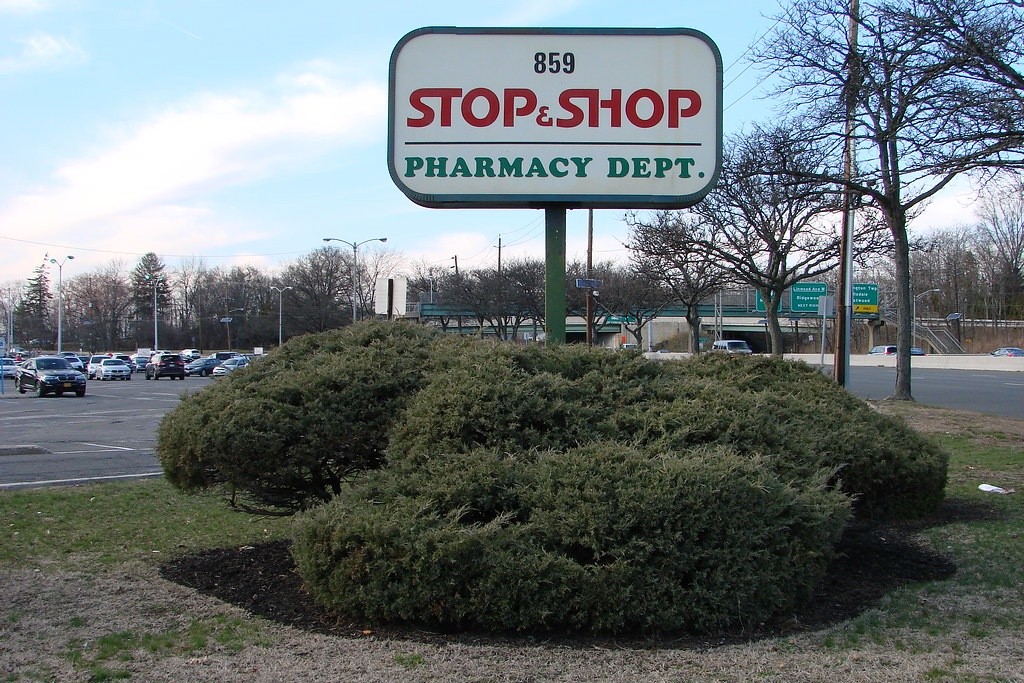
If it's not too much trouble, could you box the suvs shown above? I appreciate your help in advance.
[867,345,897,354]
[208,351,268,358]
[146,354,184,380]
[179,348,201,359]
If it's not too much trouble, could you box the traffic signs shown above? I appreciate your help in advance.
[852,283,878,313]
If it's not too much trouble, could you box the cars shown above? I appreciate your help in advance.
[991,346,1024,357]
[910,347,929,355]
[184,358,222,376]
[15,356,86,398]
[97,359,131,381]
[212,358,251,376]
[0,352,57,379]
[61,352,150,373]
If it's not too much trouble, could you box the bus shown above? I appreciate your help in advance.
[622,344,637,352]
[711,340,753,355]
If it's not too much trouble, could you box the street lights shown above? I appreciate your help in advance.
[0,286,27,355]
[146,274,171,354]
[913,288,941,346]
[51,256,75,357]
[228,308,245,351]
[271,286,293,347]
[324,237,387,325]
